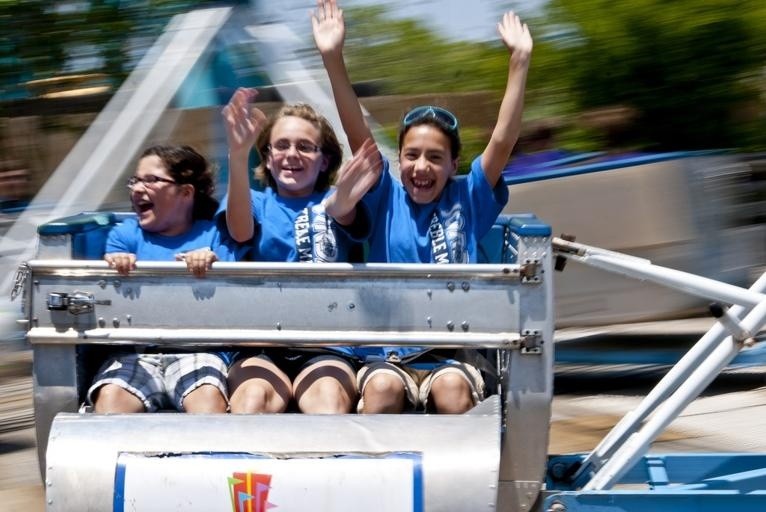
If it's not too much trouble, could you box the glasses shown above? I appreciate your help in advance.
[267,142,319,153]
[127,175,175,186]
[404,106,457,129]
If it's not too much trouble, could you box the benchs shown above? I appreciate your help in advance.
[23,210,554,512]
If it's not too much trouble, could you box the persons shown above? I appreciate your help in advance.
[214,87,383,414]
[84,144,237,414]
[308,0,535,414]
